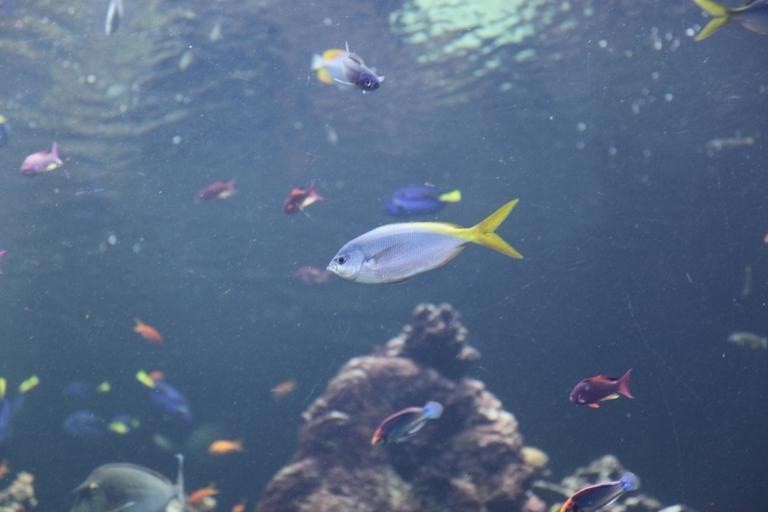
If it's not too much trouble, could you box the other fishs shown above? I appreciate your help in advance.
[0,318,297,512]
